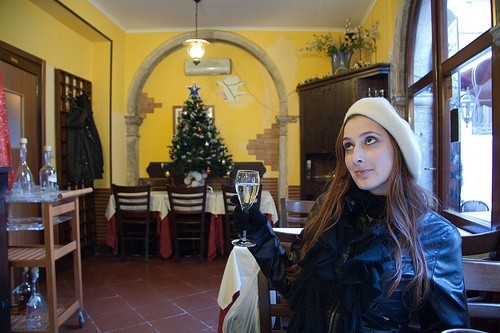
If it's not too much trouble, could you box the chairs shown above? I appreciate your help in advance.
[281,198,314,228]
[112,183,261,261]
[257,269,293,333]
[459,227,500,333]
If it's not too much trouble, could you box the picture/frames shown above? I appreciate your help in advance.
[173,106,214,140]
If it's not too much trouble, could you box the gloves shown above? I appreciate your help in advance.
[230,195,267,238]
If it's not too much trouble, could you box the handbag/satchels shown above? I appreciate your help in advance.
[218,267,262,333]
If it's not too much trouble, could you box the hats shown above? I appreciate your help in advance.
[344,96,422,179]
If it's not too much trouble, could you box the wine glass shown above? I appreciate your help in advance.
[231,170,261,248]
[25,266,49,329]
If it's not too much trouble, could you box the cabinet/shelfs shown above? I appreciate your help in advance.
[296,63,390,227]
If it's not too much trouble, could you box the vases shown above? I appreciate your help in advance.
[332,52,352,75]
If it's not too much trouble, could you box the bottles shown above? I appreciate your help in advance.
[17,267,32,315]
[39,146,58,200]
[12,137,36,200]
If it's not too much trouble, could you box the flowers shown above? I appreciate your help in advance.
[300,18,380,57]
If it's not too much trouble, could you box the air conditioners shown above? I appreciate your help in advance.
[184,59,231,75]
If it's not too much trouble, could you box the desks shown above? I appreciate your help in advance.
[273,228,304,243]
[105,191,278,261]
[8,187,93,333]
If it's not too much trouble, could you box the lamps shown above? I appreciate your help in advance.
[183,0,210,66]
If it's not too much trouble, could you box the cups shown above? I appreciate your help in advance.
[7,217,42,229]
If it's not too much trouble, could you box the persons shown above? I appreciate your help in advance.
[230,97,469,333]
